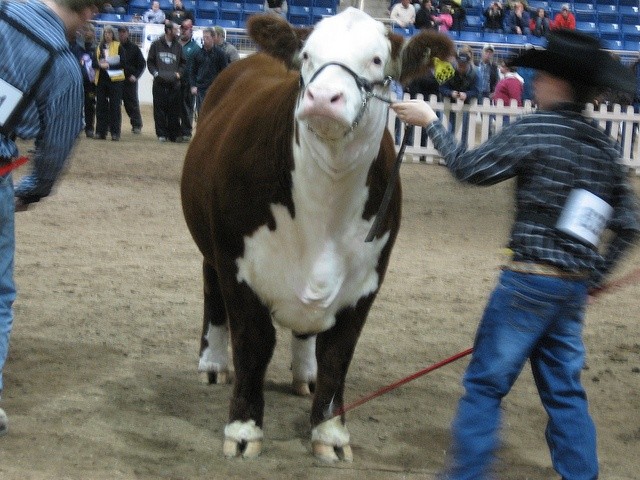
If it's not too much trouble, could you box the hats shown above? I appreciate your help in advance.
[505,27,638,92]
[483,44,495,52]
[457,46,472,63]
[118,25,129,31]
[178,19,194,29]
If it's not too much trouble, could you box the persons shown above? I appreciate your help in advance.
[415,0,433,30]
[431,7,453,32]
[437,45,484,167]
[146,21,188,142]
[445,2,469,31]
[474,44,498,104]
[167,0,195,27]
[264,0,288,23]
[488,59,525,129]
[211,28,240,69]
[190,29,225,117]
[0,0,106,431]
[483,2,503,33]
[407,79,439,162]
[70,31,99,138]
[127,13,145,47]
[412,0,426,26]
[504,0,532,36]
[529,7,551,35]
[389,0,415,30]
[173,19,212,141]
[144,1,165,25]
[108,26,147,134]
[388,25,638,479]
[386,77,408,146]
[93,26,127,141]
[103,0,132,19]
[551,5,576,32]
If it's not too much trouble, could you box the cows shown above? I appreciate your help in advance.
[181,6,458,465]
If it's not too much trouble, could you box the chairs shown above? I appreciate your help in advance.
[96,1,337,36]
[393,1,639,58]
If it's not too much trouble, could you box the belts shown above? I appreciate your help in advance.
[508,260,589,278]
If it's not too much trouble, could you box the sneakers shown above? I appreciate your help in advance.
[183,134,192,142]
[134,127,141,134]
[112,134,119,140]
[92,134,106,139]
[86,130,93,137]
[158,136,167,142]
[171,136,183,142]
